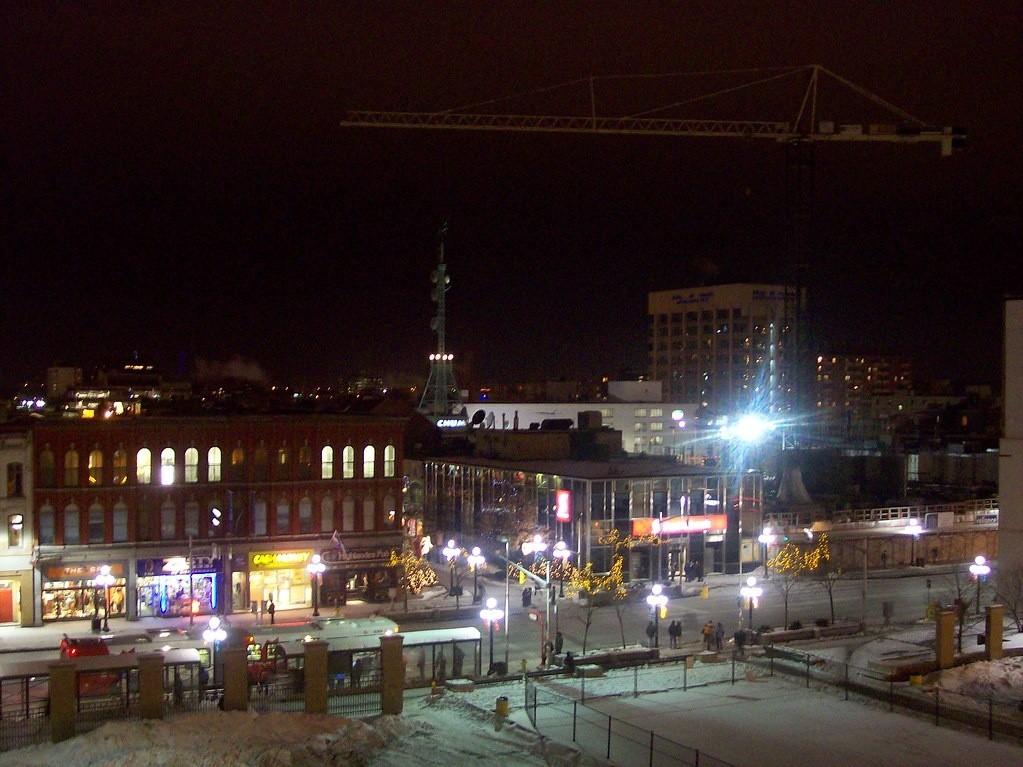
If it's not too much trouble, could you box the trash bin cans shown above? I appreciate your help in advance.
[91,617,101,633]
[496,697,508,717]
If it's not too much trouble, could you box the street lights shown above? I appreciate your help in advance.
[647,583,668,649]
[904,518,922,567]
[526,535,547,589]
[466,546,486,605]
[306,554,326,616]
[94,564,115,632]
[740,575,764,628]
[968,555,990,616]
[203,615,229,690]
[479,598,505,676]
[441,539,461,597]
[757,527,775,579]
[552,540,571,598]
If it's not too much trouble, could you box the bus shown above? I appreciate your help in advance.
[55,633,213,699]
[238,613,399,687]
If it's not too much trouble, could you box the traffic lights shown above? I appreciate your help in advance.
[782,527,790,542]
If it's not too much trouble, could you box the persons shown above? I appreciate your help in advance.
[540,636,553,664]
[417,648,425,680]
[670,561,677,581]
[646,621,656,647]
[453,645,465,676]
[733,630,746,655]
[351,659,364,689]
[434,650,446,672]
[552,586,556,605]
[703,621,713,644]
[174,673,183,697]
[269,600,274,624]
[522,587,531,606]
[882,550,888,568]
[685,560,704,582]
[200,666,208,689]
[669,620,681,649]
[554,632,562,654]
[564,651,574,672]
[74,589,89,609]
[114,587,124,612]
[715,623,723,650]
